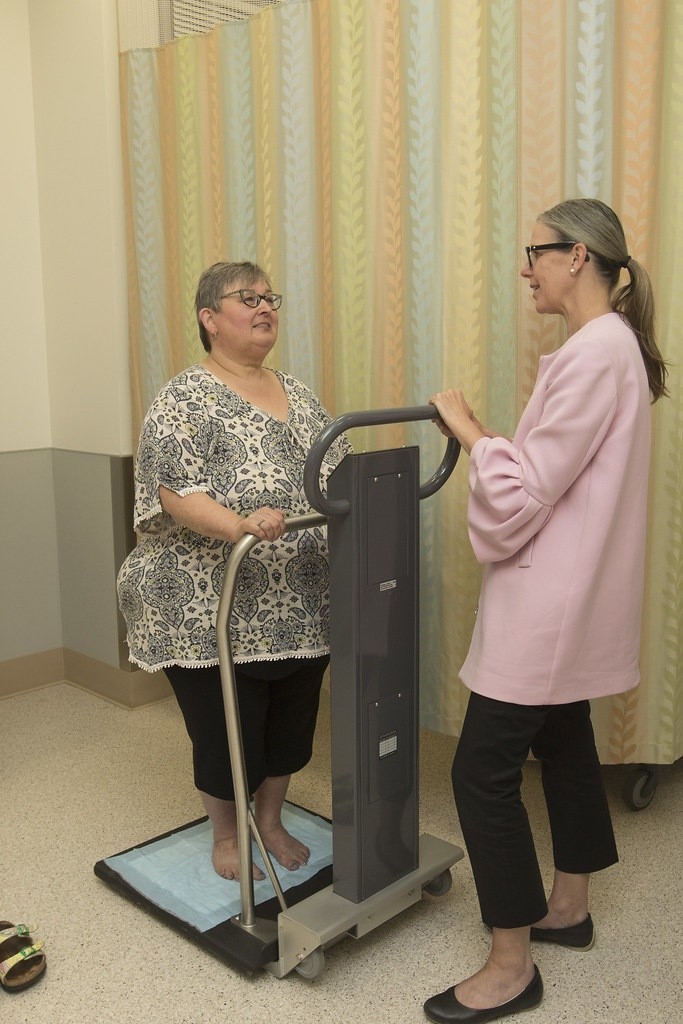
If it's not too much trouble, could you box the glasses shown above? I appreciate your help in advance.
[220,288,282,310]
[526,242,590,267]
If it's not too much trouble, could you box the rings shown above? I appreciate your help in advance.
[257,518,265,527]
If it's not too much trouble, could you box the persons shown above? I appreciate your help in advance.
[424,199,673,1023]
[117,260,353,882]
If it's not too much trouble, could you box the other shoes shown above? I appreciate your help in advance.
[424,964,544,1024]
[486,912,595,952]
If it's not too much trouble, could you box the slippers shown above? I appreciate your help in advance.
[0,920,47,992]
